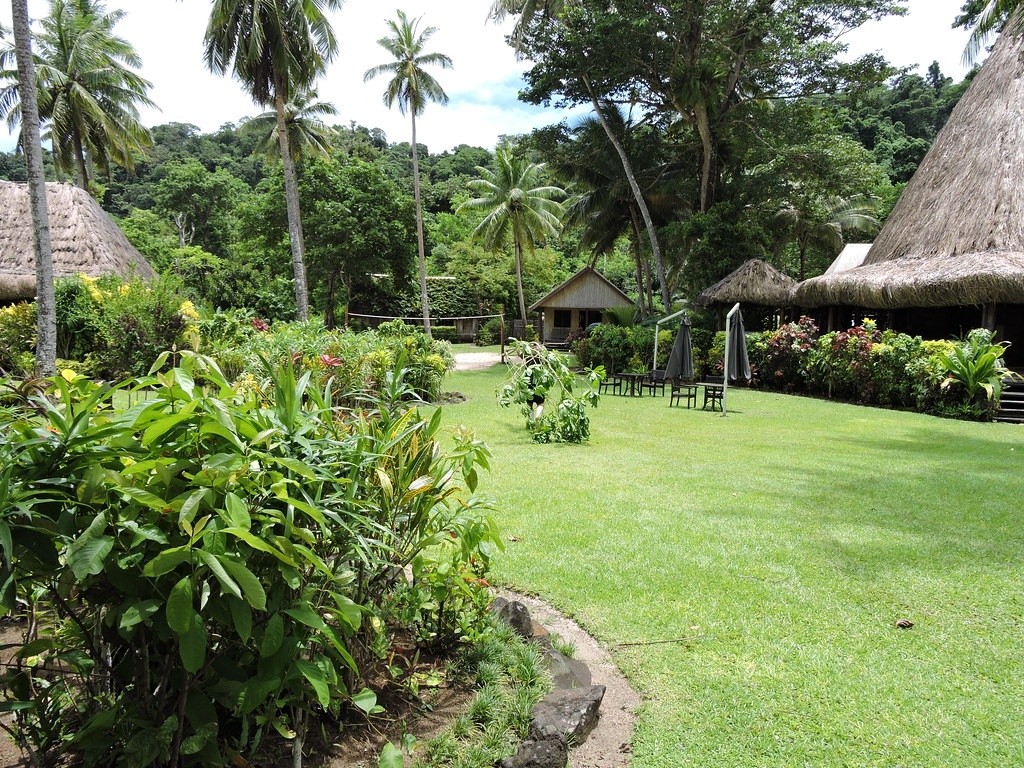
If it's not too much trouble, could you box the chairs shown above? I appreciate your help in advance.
[703,375,726,412]
[598,367,623,395]
[670,377,699,409]
[640,369,666,397]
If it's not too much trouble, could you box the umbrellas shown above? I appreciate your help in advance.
[728,309,751,381]
[663,315,694,381]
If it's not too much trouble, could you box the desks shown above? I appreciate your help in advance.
[695,383,734,411]
[617,373,651,397]
